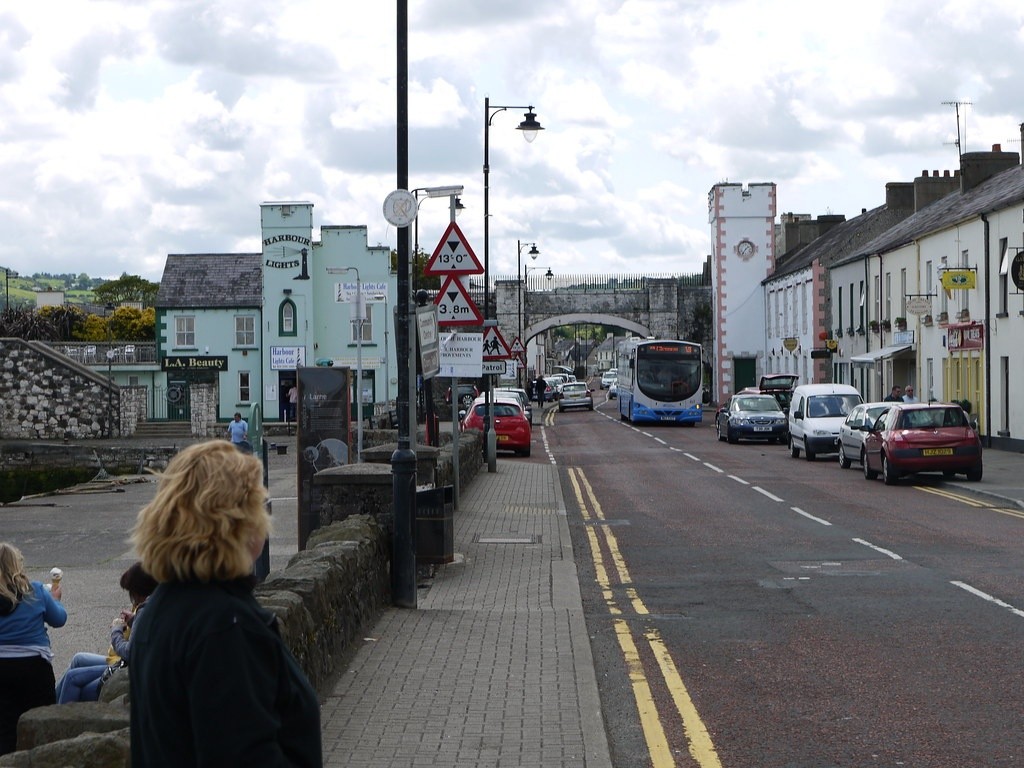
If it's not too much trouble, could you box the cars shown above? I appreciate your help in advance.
[447,385,480,407]
[459,387,534,457]
[716,394,789,443]
[857,401,983,485]
[600,367,618,390]
[532,373,577,403]
[713,372,800,429]
[607,379,617,400]
[366,410,397,428]
[836,402,906,469]
[557,382,595,411]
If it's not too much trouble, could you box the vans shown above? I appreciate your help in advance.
[786,383,864,461]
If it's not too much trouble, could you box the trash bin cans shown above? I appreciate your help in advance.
[414,483,455,565]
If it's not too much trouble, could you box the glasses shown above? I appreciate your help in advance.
[905,389,913,392]
[262,498,272,515]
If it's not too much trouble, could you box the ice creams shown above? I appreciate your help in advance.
[50,567,63,590]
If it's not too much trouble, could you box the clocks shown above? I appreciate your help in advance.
[737,241,755,259]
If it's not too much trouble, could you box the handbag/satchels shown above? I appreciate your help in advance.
[97,659,126,689]
[546,383,551,392]
[240,440,249,450]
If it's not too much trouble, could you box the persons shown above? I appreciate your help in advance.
[227,412,248,445]
[56,561,159,705]
[525,376,547,408]
[0,542,67,756]
[286,384,297,421]
[128,440,324,768]
[884,385,918,403]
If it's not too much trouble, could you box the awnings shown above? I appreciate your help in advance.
[850,343,912,369]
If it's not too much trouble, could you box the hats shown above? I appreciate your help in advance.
[120,562,157,592]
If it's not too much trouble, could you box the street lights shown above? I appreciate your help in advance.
[374,291,389,421]
[517,239,541,387]
[106,350,114,444]
[481,95,546,458]
[407,183,468,453]
[325,263,362,461]
[524,264,554,382]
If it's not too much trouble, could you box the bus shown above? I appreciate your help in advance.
[615,336,710,427]
